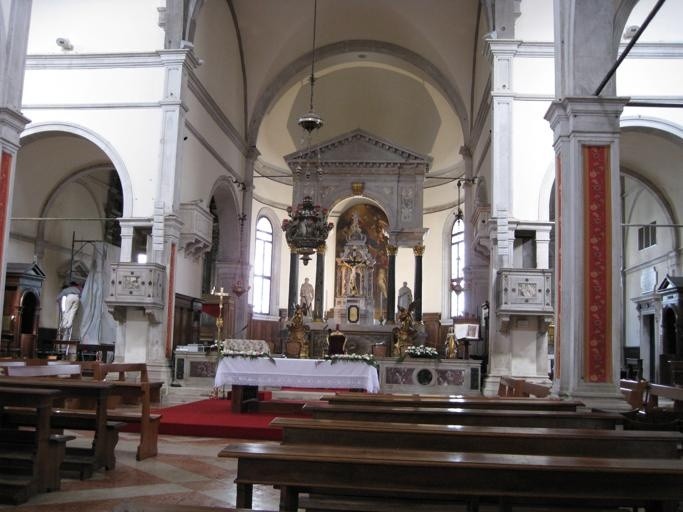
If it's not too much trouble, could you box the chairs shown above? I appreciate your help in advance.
[326,330,348,354]
[284,341,302,358]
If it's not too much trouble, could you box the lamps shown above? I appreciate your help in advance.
[280,1,334,270]
[226,176,251,297]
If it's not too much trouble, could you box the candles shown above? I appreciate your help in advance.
[219,286,224,306]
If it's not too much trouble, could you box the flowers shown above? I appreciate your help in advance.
[395,344,443,364]
[318,352,379,370]
[217,349,271,360]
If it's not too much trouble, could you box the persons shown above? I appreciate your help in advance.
[300,277,314,316]
[397,282,412,313]
[56,282,80,330]
[343,261,362,287]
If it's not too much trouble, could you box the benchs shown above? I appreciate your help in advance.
[217,392,682,509]
[0,334,164,506]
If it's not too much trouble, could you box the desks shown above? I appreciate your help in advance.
[172,350,220,383]
